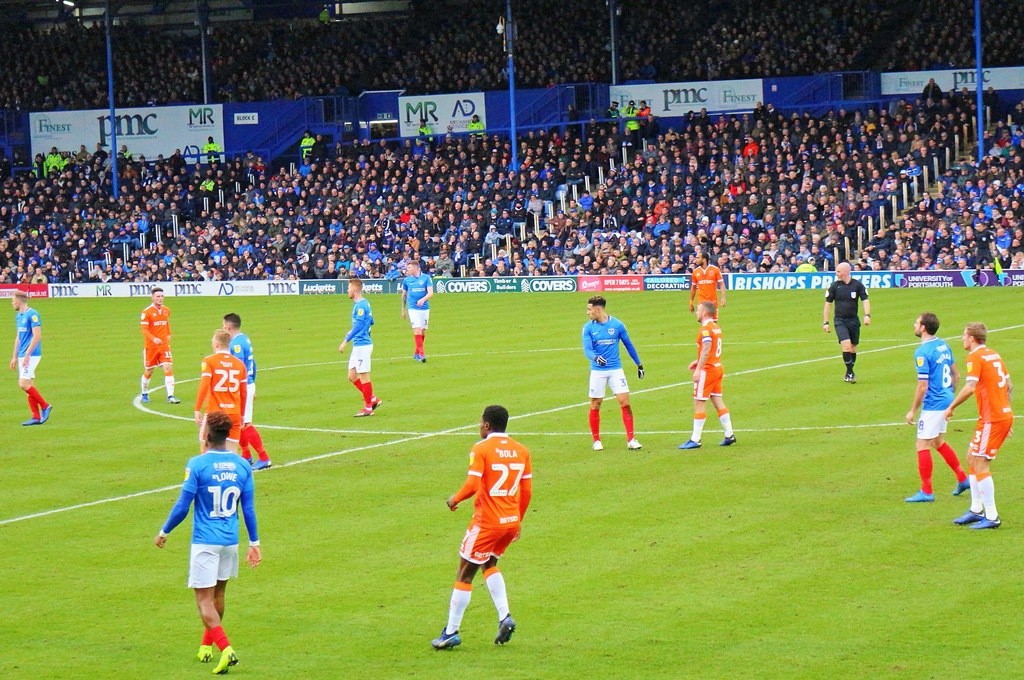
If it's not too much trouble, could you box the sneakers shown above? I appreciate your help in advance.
[952,477,970,495]
[369,393,382,409]
[968,517,1001,530]
[846,373,856,382]
[678,438,701,449]
[905,490,936,503]
[40,404,52,424]
[492,612,517,645]
[212,644,239,675]
[626,438,642,449]
[431,627,461,647]
[955,510,986,525]
[248,457,272,470]
[844,371,850,381]
[592,440,604,450]
[196,644,212,663]
[21,418,39,426]
[167,395,182,404]
[719,434,737,445]
[353,406,374,418]
[141,393,150,403]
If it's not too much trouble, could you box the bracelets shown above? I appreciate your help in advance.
[824,321,829,325]
[865,314,870,318]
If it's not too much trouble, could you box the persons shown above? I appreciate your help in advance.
[193,328,248,455]
[140,287,182,404]
[678,300,737,450]
[0,0,1024,284]
[904,311,971,503]
[943,321,1016,531]
[430,404,533,650]
[401,260,434,363]
[822,262,872,384]
[581,295,645,451]
[154,411,263,674]
[338,279,382,417]
[9,291,53,426]
[222,313,272,471]
[688,251,727,325]
